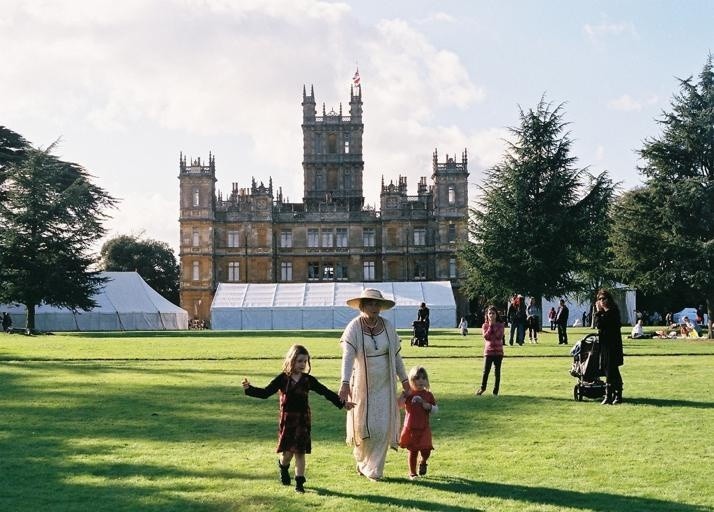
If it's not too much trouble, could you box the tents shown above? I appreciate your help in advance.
[211,280,457,330]
[0,269,189,330]
[541,269,637,327]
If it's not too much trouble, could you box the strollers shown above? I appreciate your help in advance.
[568,333,606,399]
[410,320,429,346]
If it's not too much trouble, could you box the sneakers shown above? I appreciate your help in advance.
[419,463,427,474]
[410,473,418,480]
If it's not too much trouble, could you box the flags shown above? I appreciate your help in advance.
[354,69,360,86]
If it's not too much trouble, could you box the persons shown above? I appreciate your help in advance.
[338,289,409,481]
[582,311,586,327]
[242,344,356,494]
[475,306,505,395]
[586,311,592,326]
[417,303,430,346]
[496,294,570,346]
[629,304,706,339]
[398,367,439,480]
[459,317,468,335]
[595,291,623,405]
[0,312,11,331]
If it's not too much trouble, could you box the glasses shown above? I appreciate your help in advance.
[598,296,608,300]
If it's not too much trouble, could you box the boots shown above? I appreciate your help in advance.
[600,385,622,405]
[294,475,306,494]
[279,459,291,485]
[529,337,538,344]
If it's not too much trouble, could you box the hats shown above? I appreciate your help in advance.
[346,288,395,310]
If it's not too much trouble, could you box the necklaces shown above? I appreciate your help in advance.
[362,314,381,350]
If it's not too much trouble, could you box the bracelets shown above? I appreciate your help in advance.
[401,380,409,383]
[342,381,349,384]
